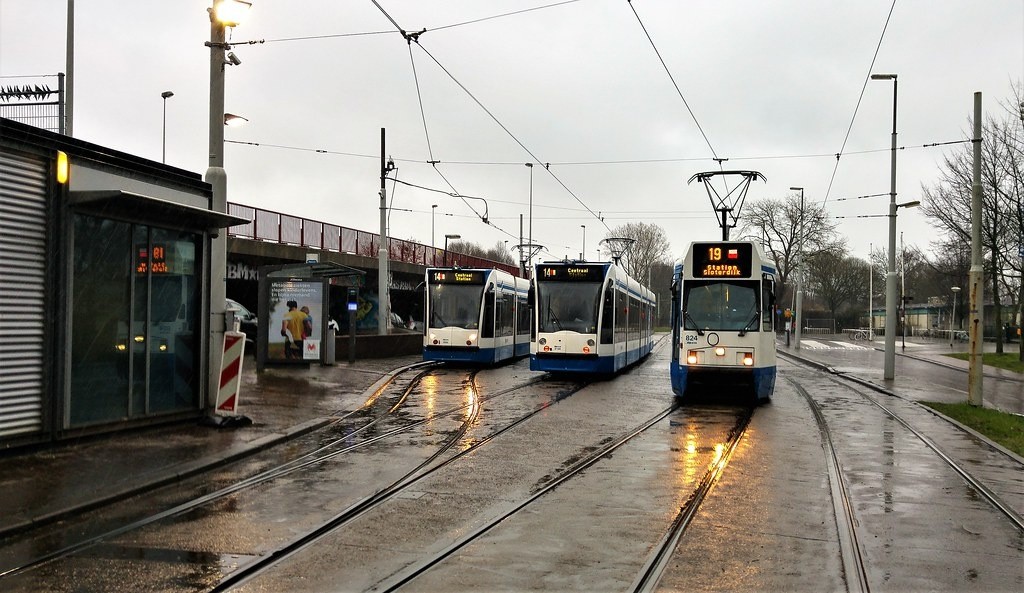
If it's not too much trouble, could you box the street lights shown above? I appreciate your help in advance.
[597,249,600,262]
[504,241,508,262]
[580,225,586,263]
[161,91,174,165]
[871,74,920,380]
[431,204,438,265]
[790,186,804,350]
[443,234,461,267]
[525,162,533,279]
[949,286,961,349]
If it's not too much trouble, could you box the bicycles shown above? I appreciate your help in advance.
[849,328,876,341]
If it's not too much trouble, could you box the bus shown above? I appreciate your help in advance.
[670,158,778,402]
[526,237,657,377]
[423,244,548,365]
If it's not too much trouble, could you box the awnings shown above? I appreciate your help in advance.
[70,190,251,237]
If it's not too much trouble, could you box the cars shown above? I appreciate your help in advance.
[221,297,261,352]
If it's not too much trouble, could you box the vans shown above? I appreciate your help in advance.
[113,241,196,358]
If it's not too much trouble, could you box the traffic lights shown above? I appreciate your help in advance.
[785,308,790,318]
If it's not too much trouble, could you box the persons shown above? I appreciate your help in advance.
[281,300,312,359]
